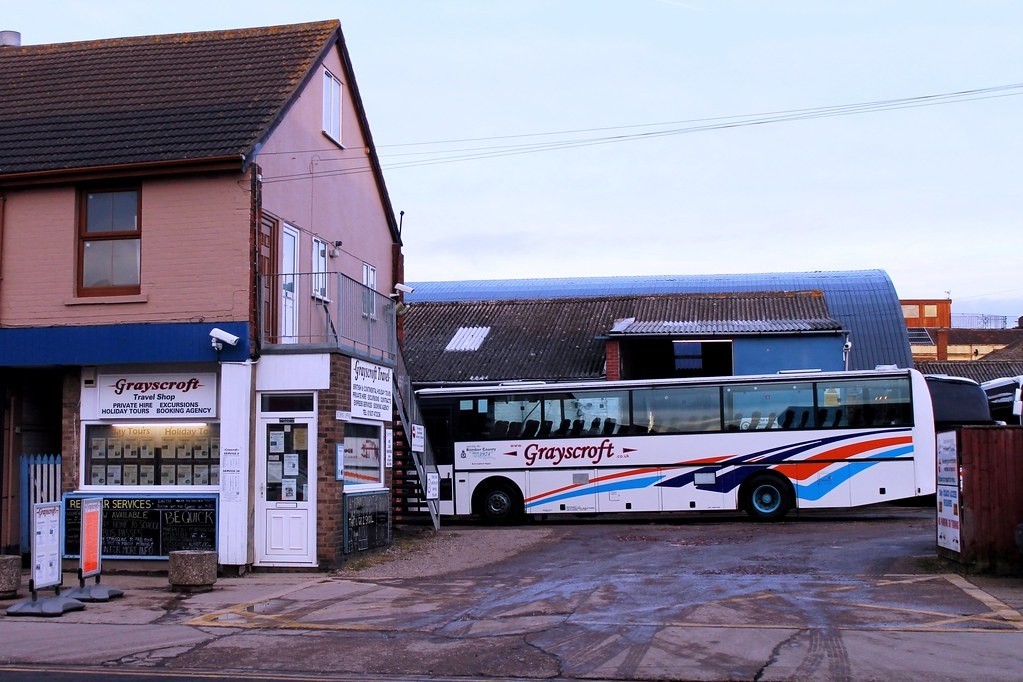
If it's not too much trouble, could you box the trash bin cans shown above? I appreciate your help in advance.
[935,424,1023,567]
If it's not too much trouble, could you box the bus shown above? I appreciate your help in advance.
[403,364,937,528]
[979,376,1023,426]
[922,373,992,434]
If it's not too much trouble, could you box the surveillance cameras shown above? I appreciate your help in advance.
[844,342,852,352]
[390,283,415,298]
[210,328,240,350]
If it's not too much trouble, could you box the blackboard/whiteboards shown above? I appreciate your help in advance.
[61,493,219,560]
[343,490,389,554]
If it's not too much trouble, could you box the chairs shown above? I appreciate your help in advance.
[486,406,910,441]
